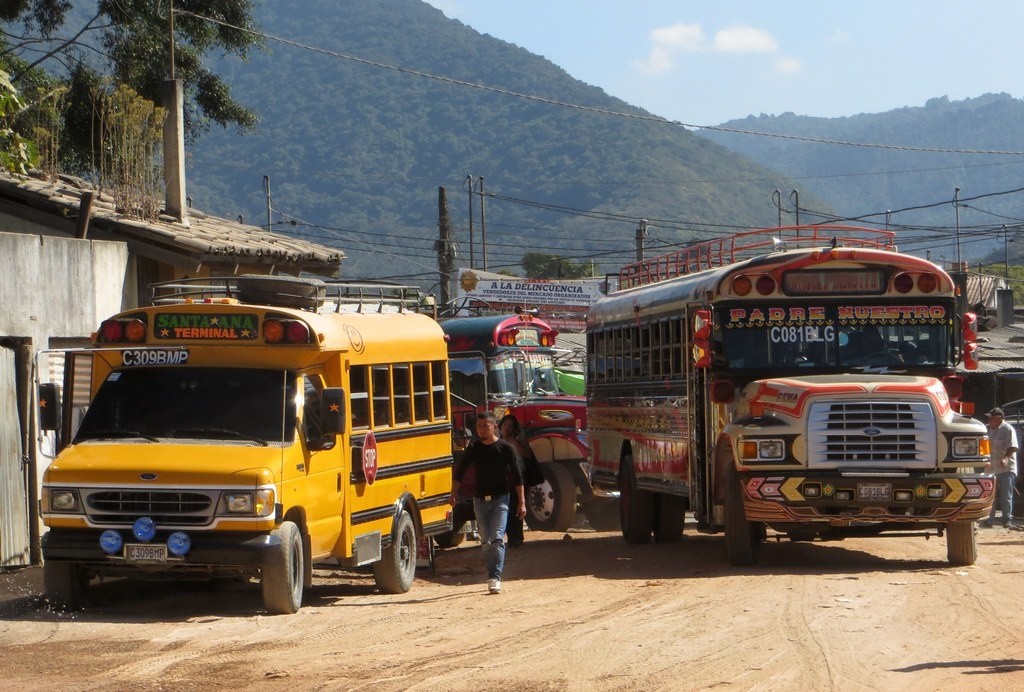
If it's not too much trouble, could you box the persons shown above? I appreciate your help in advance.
[449,411,526,594]
[499,414,532,548]
[452,427,481,541]
[981,408,1019,527]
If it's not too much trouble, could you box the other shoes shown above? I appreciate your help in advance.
[979,522,991,528]
[1003,523,1010,528]
[489,579,500,594]
[507,539,522,548]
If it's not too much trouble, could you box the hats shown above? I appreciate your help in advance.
[985,407,1004,418]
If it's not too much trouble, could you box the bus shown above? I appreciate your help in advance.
[34,276,455,614]
[438,295,621,531]
[584,225,997,566]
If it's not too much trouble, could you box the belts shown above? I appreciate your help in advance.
[474,493,506,501]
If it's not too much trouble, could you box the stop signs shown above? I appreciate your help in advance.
[362,431,379,487]
